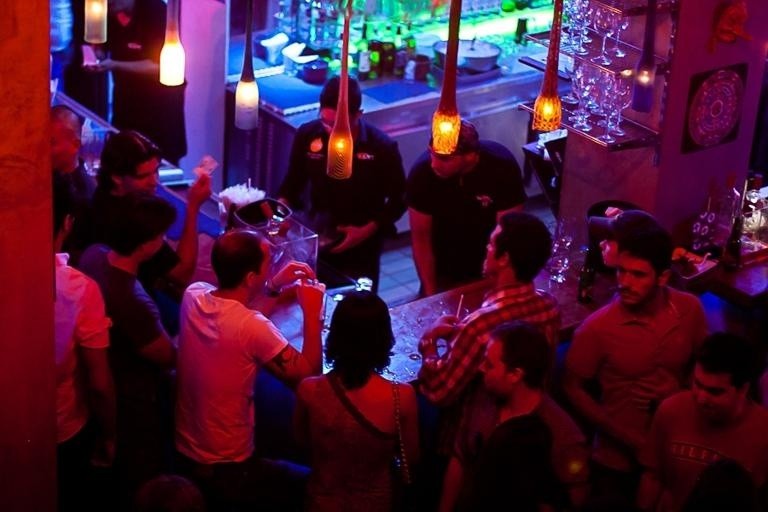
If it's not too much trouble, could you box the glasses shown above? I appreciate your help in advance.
[317,108,364,129]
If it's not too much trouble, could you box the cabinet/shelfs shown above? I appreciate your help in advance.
[514,1,676,153]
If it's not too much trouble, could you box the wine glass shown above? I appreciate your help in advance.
[742,173,768,253]
[545,217,582,291]
[559,1,638,147]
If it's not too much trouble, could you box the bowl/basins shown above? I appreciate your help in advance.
[431,39,501,74]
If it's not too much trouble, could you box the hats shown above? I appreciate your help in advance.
[587,210,661,241]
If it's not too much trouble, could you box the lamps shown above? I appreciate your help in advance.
[233,1,261,129]
[323,1,360,178]
[532,0,567,134]
[425,1,466,155]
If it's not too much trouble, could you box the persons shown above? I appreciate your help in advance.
[274,78,408,292]
[79,190,178,512]
[397,121,531,298]
[52,181,124,512]
[51,104,94,224]
[52,211,767,512]
[70,122,214,321]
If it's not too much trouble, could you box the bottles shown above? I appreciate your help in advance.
[212,194,296,276]
[334,18,430,83]
[722,216,743,272]
[578,251,595,303]
